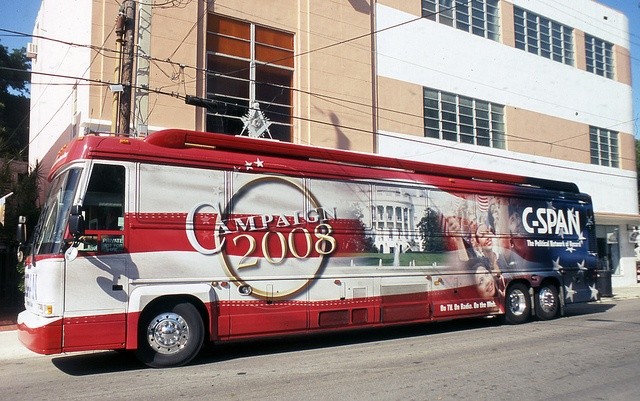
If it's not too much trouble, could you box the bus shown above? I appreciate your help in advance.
[16,129,600,369]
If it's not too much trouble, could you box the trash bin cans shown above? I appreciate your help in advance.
[598,270,613,297]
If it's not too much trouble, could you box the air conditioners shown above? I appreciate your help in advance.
[26,42,38,58]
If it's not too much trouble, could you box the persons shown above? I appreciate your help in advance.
[78,215,120,243]
[446,197,536,314]
